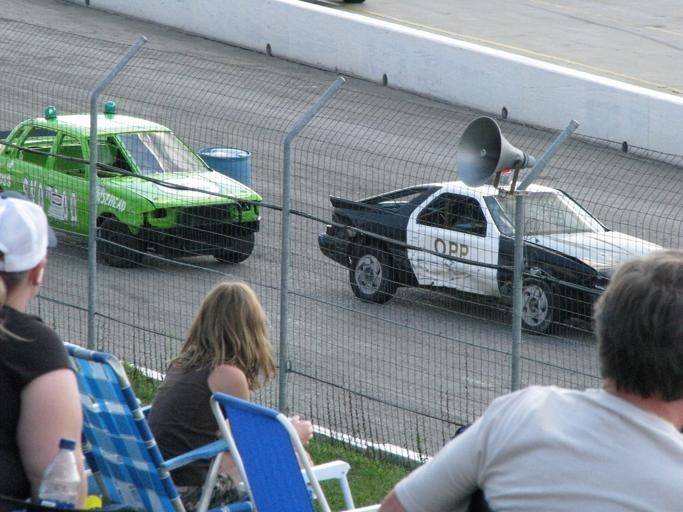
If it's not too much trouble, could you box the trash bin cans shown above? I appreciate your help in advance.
[198,146,251,188]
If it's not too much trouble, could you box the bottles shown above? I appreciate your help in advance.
[36,438,79,509]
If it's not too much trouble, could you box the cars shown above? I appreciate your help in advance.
[0,100,263,269]
[317,178,667,336]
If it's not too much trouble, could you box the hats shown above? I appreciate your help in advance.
[0,190,57,274]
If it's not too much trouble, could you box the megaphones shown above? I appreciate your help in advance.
[456,115,536,187]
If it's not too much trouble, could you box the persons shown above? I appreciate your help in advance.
[0,194,90,511]
[373,246,681,512]
[146,279,316,512]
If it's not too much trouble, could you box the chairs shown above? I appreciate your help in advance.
[210,392,381,512]
[62,340,228,511]
